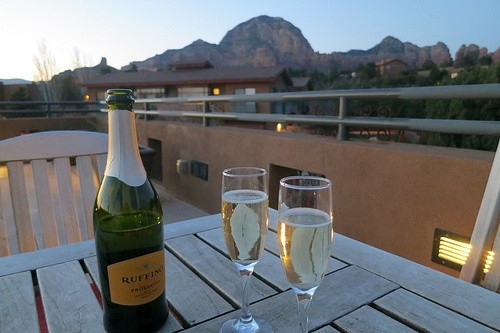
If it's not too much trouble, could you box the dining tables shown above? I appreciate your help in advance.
[0,205,500,333]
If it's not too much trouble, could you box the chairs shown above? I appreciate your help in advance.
[0,131,108,257]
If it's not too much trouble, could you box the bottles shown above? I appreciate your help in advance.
[92,89,169,333]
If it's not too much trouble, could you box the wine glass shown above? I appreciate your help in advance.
[220,166,275,333]
[277,176,334,333]
